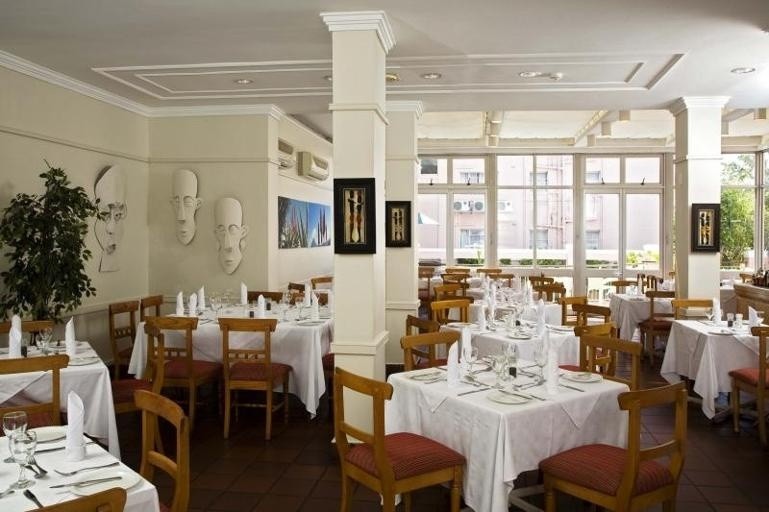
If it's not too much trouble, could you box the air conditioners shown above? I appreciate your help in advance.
[453,199,471,212]
[300,154,330,181]
[472,201,485,211]
[278,139,295,168]
[498,201,511,213]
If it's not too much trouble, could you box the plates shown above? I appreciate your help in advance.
[508,329,532,340]
[67,354,99,367]
[708,327,736,335]
[33,426,65,444]
[49,339,80,349]
[563,372,602,384]
[487,386,531,406]
[71,467,140,498]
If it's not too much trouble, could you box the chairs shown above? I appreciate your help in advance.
[434,285,460,298]
[219,318,292,441]
[135,391,190,512]
[109,301,139,384]
[0,355,69,437]
[740,274,759,282]
[1,320,51,348]
[540,382,688,512]
[331,367,466,512]
[578,336,639,397]
[646,274,659,291]
[558,297,588,326]
[530,275,565,302]
[313,277,333,289]
[573,302,612,330]
[639,291,676,370]
[574,322,616,376]
[730,327,769,448]
[408,316,440,366]
[477,269,502,278]
[614,281,638,294]
[109,322,165,455]
[141,296,163,327]
[441,274,470,288]
[401,332,462,371]
[445,269,471,277]
[489,275,514,289]
[419,269,434,309]
[430,299,468,327]
[145,318,223,433]
[671,300,713,323]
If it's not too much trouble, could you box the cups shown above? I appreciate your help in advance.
[735,312,744,327]
[726,312,734,327]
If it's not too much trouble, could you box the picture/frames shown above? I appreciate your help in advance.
[333,178,376,256]
[689,203,719,254]
[386,201,411,249]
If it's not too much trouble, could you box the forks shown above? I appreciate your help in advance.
[200,319,213,325]
[52,460,120,476]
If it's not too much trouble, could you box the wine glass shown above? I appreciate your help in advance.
[461,346,477,380]
[308,290,319,305]
[294,296,305,320]
[282,289,293,307]
[2,409,27,464]
[532,348,548,384]
[38,326,53,351]
[185,292,199,309]
[248,302,254,317]
[210,289,229,322]
[500,342,519,365]
[7,431,37,492]
[264,297,271,314]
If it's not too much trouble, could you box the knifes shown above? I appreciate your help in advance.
[458,385,490,398]
[49,476,124,490]
[558,381,585,392]
